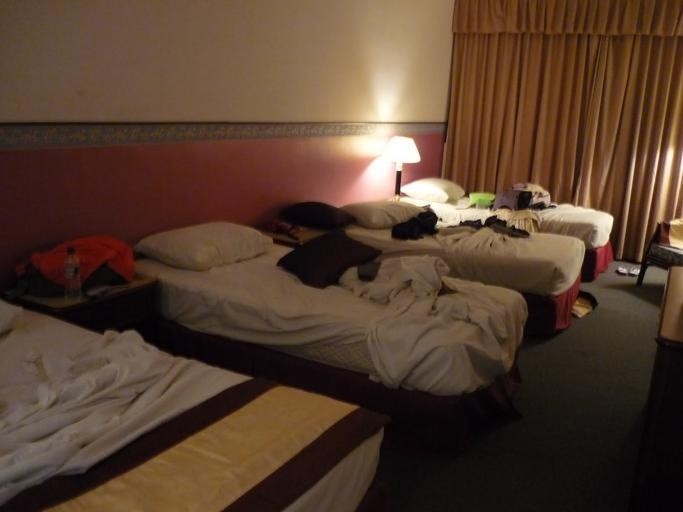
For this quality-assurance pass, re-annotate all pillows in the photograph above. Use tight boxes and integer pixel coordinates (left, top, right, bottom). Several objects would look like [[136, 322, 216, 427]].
[[273, 228, 383, 291], [401, 178, 467, 202], [340, 201, 425, 230], [132, 221, 273, 275], [285, 200, 355, 229], [0, 298, 25, 334]]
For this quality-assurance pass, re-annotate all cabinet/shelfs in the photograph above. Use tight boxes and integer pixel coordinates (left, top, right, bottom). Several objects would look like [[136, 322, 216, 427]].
[[630, 264, 683, 512]]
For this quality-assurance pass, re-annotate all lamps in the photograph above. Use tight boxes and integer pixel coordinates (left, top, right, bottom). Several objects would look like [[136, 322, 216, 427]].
[[379, 137, 421, 199]]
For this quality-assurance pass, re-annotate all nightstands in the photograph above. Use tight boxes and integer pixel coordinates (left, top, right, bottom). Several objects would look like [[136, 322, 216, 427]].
[[4, 272, 160, 345], [266, 219, 328, 246]]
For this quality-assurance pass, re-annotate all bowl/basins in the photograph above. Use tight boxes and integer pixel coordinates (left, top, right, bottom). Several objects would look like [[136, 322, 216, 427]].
[[468, 193, 495, 208]]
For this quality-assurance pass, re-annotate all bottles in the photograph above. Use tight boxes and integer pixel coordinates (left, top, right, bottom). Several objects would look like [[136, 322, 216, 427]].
[[64, 247, 81, 303]]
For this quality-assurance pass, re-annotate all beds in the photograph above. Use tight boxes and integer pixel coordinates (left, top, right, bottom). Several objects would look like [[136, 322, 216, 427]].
[[335, 200, 584, 343], [399, 172, 617, 282], [0, 298, 390, 510], [132, 218, 532, 447]]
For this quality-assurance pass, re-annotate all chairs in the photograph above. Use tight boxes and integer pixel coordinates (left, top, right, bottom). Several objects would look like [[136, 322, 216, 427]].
[[635, 219, 683, 285]]
